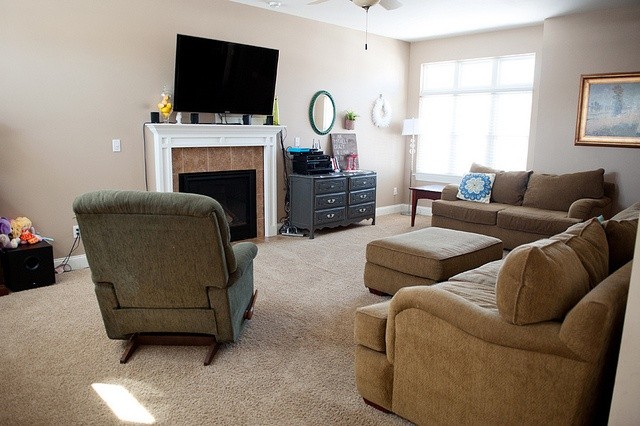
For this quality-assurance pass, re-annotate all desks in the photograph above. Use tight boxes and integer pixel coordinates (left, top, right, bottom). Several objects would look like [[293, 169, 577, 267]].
[[409, 185, 446, 227]]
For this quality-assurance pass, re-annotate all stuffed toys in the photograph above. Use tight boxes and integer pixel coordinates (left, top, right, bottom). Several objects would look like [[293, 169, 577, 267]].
[[17, 216, 38, 243], [0, 219, 22, 248]]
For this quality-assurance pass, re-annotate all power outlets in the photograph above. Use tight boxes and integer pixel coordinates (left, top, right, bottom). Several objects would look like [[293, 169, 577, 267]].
[[73, 226, 82, 238], [393, 186, 398, 195]]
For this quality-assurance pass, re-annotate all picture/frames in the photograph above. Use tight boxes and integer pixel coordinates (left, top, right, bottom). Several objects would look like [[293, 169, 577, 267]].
[[574, 71, 640, 148]]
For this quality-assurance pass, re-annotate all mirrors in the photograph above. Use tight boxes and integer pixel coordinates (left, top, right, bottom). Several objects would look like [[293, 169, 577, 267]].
[[308, 89, 336, 136]]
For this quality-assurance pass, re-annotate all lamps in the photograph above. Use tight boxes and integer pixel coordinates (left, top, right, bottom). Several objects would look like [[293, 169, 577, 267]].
[[401, 119, 420, 216], [350, 0, 380, 50]]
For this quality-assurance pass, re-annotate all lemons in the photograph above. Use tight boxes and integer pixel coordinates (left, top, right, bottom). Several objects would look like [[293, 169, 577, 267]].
[[158, 96, 172, 118]]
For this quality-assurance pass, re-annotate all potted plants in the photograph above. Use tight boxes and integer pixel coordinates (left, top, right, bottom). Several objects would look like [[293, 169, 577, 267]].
[[344, 111, 360, 130]]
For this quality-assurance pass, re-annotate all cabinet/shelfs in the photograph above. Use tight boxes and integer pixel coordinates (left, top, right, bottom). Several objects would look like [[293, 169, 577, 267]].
[[348, 170, 377, 225], [288, 172, 348, 239]]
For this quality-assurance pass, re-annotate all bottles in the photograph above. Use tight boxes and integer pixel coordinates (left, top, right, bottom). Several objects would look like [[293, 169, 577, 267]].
[[273, 98, 279, 123]]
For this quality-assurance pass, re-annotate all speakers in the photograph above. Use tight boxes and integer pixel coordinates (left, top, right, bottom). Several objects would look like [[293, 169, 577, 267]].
[[0, 239, 56, 292]]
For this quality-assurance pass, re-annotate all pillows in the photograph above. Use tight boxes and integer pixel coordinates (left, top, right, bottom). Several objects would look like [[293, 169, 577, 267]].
[[601, 201, 639, 260], [523, 168, 605, 212], [456, 173, 496, 204], [495, 218, 609, 326], [469, 163, 533, 206]]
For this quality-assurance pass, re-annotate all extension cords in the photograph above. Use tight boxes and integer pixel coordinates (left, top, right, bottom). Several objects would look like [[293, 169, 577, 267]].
[[281, 232, 305, 237]]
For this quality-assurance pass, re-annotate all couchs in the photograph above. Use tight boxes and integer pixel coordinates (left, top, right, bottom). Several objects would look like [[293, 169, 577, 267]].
[[364, 227, 503, 297], [431, 182, 619, 250], [355, 259, 632, 426], [72, 189, 258, 366]]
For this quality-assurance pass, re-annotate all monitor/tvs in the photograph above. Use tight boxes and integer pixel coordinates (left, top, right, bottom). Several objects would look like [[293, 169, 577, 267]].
[[173, 33, 279, 125]]
